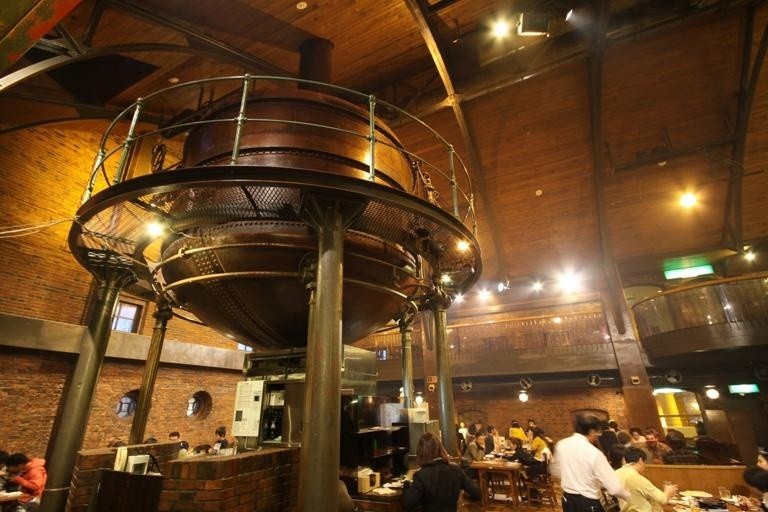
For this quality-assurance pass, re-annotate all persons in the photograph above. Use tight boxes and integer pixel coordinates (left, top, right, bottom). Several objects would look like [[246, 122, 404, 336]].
[[0, 451, 48, 512], [401, 432, 482, 512], [742, 451, 768, 512], [547, 414, 678, 512], [456, 418, 555, 504], [664, 430, 732, 465], [107, 427, 240, 454]]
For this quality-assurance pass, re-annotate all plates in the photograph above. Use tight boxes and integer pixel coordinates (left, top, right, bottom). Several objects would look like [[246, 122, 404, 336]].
[[680, 490, 712, 498], [383, 482, 403, 488]]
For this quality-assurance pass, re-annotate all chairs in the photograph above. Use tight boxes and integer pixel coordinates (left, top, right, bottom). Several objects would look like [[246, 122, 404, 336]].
[[527, 460, 559, 510]]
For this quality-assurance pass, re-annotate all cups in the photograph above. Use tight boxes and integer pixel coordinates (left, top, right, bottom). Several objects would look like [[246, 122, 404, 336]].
[[661, 480, 672, 486], [717, 486, 730, 498]]
